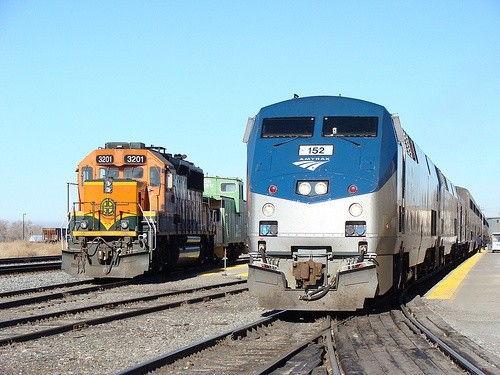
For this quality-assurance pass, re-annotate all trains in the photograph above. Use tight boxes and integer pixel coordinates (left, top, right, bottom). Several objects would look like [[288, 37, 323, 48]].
[[61, 142, 248, 284], [241, 94, 489, 311]]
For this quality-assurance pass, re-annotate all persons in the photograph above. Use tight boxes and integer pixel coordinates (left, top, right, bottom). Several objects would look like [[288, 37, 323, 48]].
[[477, 235, 483, 253], [483, 235, 487, 250]]
